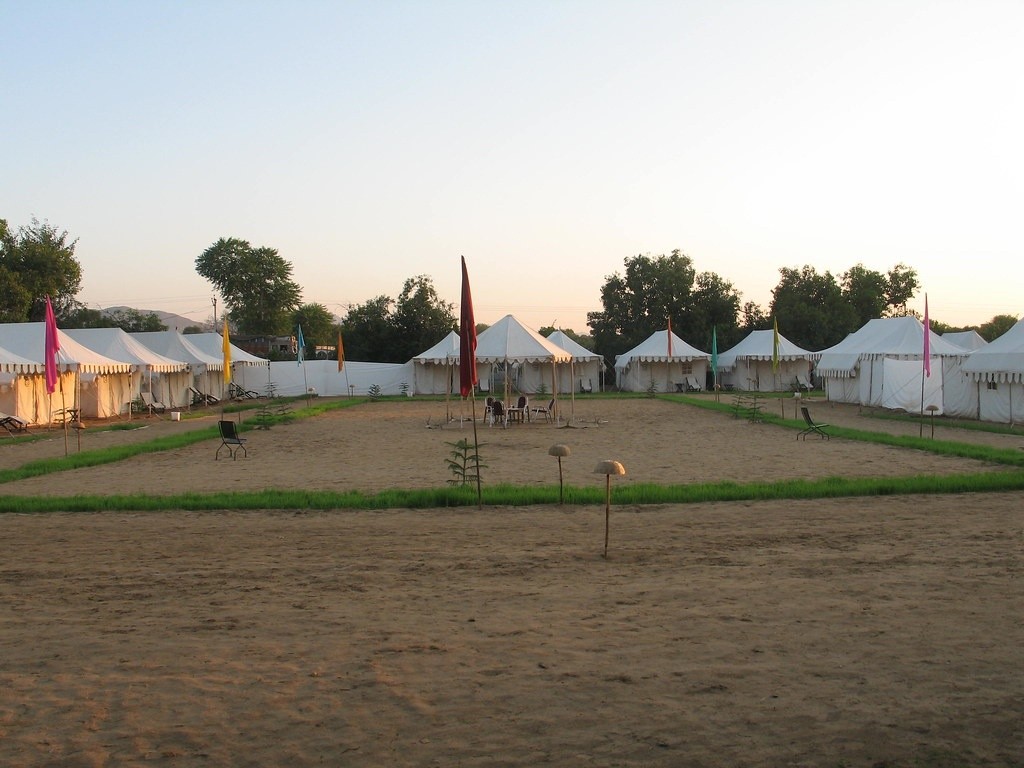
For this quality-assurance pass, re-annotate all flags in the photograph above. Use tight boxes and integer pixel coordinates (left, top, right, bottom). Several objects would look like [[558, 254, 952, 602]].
[[667, 317, 672, 358], [459, 256, 478, 398], [712, 326, 718, 375], [923, 295, 931, 377], [337, 331, 345, 373], [45, 296, 61, 394], [222, 318, 233, 386], [773, 316, 780, 373], [297, 323, 306, 367]]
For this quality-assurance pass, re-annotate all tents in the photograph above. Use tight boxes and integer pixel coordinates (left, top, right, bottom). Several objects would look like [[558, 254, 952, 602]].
[[414, 315, 605, 395], [0, 323, 271, 438], [615, 318, 1024, 426]]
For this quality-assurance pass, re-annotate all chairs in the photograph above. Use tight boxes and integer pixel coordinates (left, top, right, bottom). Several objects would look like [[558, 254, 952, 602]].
[[796, 375, 814, 392], [532, 400, 555, 423], [190, 387, 221, 407], [216, 421, 247, 461], [0, 412, 33, 439], [484, 396, 530, 428], [580, 378, 592, 392], [796, 407, 829, 441], [686, 376, 702, 393], [231, 382, 259, 399], [140, 393, 164, 414]]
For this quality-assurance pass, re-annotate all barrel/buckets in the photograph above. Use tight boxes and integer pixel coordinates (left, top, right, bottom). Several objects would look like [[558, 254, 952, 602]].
[[170, 411, 181, 421]]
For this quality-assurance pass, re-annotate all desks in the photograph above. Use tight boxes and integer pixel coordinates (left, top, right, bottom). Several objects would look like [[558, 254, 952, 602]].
[[675, 383, 683, 393], [68, 409, 82, 424], [507, 407, 524, 424], [724, 384, 733, 392]]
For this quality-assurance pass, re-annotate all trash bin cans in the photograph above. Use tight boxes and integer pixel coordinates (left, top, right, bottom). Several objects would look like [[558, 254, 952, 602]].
[[170, 411, 181, 421], [794, 392, 802, 399], [405, 390, 413, 397]]
